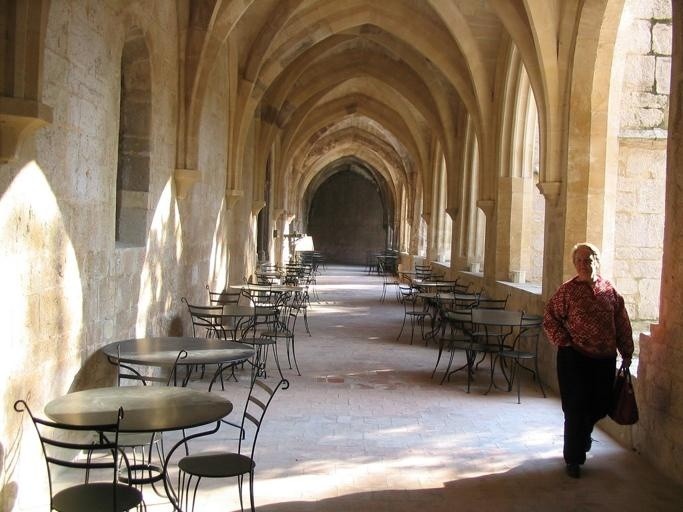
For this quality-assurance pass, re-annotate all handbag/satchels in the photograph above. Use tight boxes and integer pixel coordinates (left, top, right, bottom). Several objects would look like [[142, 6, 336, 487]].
[[607, 376, 639, 425]]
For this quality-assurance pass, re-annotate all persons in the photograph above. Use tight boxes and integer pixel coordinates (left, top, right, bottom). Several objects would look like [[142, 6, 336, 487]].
[[542, 242, 635, 479]]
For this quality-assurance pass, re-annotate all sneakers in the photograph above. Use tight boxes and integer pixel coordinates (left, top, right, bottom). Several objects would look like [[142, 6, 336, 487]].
[[562, 457, 580, 478]]
